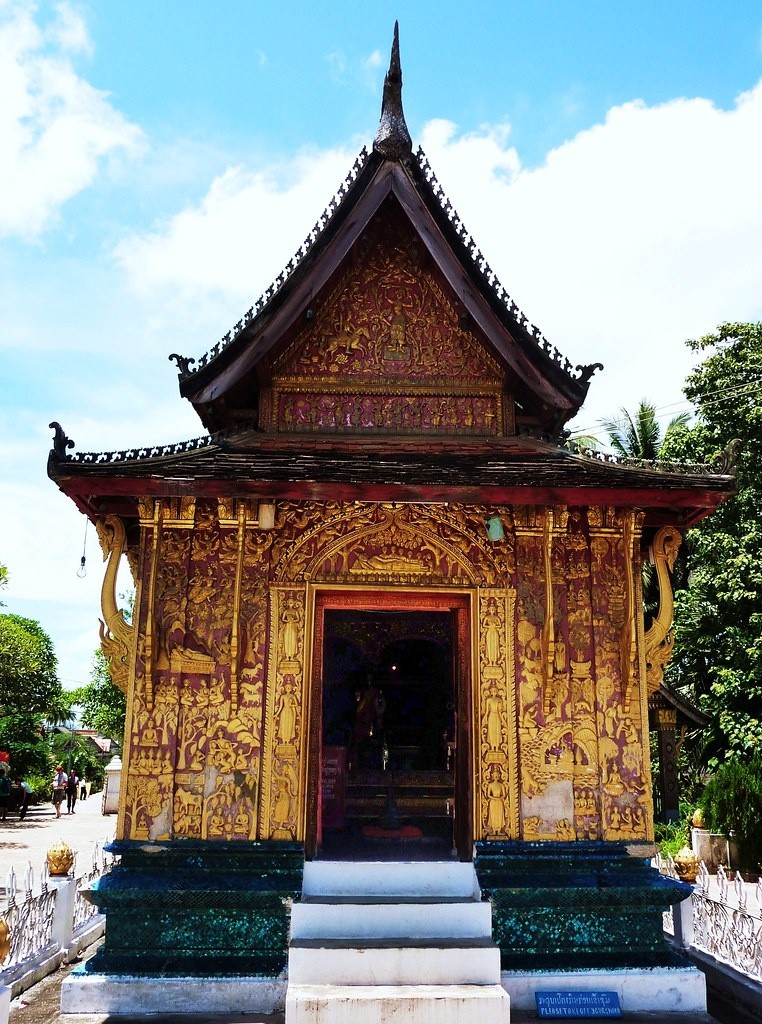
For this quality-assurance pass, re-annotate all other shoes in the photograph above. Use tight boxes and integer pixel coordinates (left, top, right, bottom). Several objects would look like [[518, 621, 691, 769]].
[[0, 818, 7, 821], [66, 811, 76, 814], [19, 816, 25, 822], [80, 798, 87, 801], [55, 814, 61, 818]]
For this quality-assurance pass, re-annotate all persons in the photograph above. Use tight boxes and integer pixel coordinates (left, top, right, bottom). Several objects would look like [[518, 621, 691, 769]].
[[384, 284, 413, 354], [483, 606, 501, 667], [282, 599, 299, 662], [483, 688, 504, 753], [15, 778, 33, 820], [52, 766, 68, 819], [487, 772, 506, 835], [66, 770, 79, 814], [271, 758, 295, 830], [0, 769, 9, 821], [80, 777, 87, 800], [274, 684, 298, 746]]
